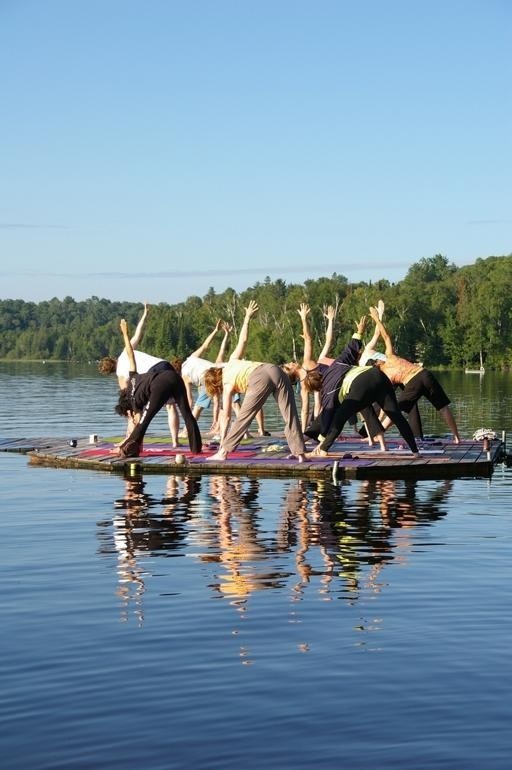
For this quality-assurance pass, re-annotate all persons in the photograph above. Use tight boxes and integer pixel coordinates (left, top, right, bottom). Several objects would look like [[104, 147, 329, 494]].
[[276, 303, 391, 452], [361, 307, 462, 446], [203, 299, 311, 463], [96, 300, 183, 450], [303, 314, 423, 458], [356, 300, 425, 440], [214, 321, 271, 436], [114, 317, 204, 457], [312, 306, 375, 448], [170, 317, 252, 439]]
